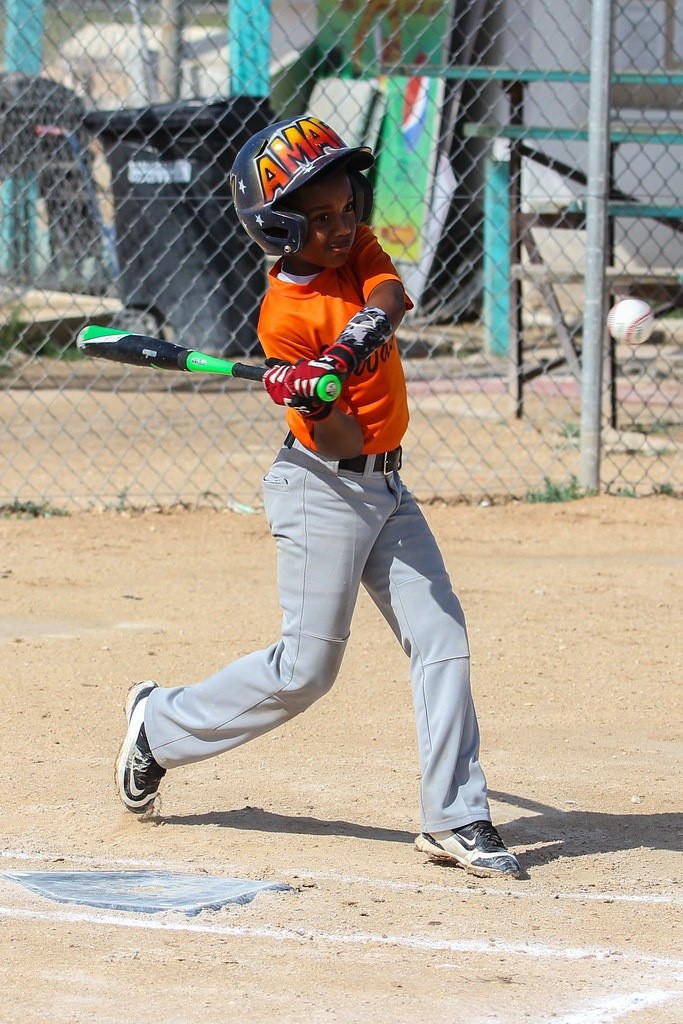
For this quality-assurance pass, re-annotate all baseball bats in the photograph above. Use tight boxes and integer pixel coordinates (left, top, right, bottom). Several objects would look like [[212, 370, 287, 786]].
[[75, 325, 344, 403]]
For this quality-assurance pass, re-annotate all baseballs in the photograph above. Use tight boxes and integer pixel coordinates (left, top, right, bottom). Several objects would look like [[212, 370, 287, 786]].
[[606, 297, 655, 346]]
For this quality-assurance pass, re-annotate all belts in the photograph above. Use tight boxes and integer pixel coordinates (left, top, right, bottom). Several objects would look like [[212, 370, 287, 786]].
[[286, 432, 404, 475]]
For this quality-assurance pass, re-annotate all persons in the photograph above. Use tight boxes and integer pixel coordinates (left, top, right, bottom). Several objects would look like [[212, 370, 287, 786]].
[[112, 113, 525, 881]]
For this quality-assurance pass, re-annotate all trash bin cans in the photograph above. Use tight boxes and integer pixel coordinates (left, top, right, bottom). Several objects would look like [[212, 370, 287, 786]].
[[83, 96, 273, 357]]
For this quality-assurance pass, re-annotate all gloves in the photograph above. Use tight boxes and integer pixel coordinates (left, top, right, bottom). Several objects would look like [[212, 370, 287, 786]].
[[285, 344, 359, 399], [261, 358, 334, 424]]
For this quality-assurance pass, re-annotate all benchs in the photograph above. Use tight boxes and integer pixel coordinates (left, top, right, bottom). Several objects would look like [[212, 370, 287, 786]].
[[412, 63, 683, 425]]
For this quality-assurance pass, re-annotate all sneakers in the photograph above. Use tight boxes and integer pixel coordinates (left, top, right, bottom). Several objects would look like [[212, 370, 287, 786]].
[[115, 681, 166, 814], [415, 819, 521, 879]]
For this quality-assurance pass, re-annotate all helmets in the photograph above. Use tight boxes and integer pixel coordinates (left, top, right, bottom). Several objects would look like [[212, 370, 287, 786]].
[[229, 115, 377, 256]]
